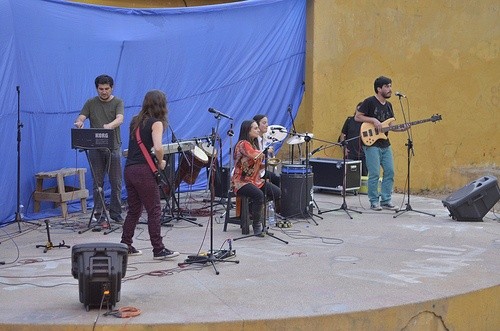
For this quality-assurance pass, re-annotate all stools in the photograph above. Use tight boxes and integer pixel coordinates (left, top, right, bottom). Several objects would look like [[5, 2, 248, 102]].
[[224, 192, 266, 235]]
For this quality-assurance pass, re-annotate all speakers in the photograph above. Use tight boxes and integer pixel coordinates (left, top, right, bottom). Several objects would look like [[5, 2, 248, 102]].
[[442, 176, 500, 222], [280, 173, 314, 220], [71, 243, 128, 310]]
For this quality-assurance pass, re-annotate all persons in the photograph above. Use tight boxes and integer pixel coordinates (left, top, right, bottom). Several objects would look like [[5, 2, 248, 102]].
[[120, 90, 180, 260], [74, 75, 125, 229], [234, 120, 281, 237], [354, 75, 399, 211], [340, 102, 368, 177], [251, 114, 281, 214]]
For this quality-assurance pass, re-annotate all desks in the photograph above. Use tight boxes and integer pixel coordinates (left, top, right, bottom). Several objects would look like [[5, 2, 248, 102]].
[[33, 167, 89, 218]]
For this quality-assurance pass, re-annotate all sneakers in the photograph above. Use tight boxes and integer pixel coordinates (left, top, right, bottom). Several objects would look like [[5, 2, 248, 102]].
[[128, 245, 142, 255], [382, 203, 398, 209], [154, 248, 179, 259], [371, 202, 382, 210]]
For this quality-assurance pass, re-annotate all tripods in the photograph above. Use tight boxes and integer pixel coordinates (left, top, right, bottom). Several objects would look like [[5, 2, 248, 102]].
[[394, 96, 435, 218], [0, 86, 42, 233], [161, 121, 362, 275]]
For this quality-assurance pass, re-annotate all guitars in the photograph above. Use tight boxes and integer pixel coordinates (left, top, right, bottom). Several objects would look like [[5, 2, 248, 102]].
[[360, 113, 442, 147], [156, 167, 171, 197]]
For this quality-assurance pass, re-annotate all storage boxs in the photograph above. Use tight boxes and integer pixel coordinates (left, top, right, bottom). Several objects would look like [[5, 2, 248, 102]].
[[309, 158, 363, 196]]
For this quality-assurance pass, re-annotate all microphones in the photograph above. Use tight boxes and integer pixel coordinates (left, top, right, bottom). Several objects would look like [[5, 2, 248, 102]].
[[208, 107, 233, 120], [267, 134, 282, 142], [395, 92, 407, 98], [308, 146, 323, 156], [271, 127, 283, 130], [287, 107, 291, 112]]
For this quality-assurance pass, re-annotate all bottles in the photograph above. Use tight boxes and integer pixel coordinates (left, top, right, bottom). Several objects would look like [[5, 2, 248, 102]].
[[19, 203, 25, 218], [269, 200, 275, 222]]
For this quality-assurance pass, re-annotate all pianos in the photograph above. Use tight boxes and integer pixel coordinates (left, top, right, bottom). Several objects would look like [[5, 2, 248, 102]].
[[122, 136, 213, 225]]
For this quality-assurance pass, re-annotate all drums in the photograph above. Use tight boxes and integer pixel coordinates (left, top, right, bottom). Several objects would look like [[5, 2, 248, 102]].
[[201, 142, 217, 168], [179, 146, 208, 184]]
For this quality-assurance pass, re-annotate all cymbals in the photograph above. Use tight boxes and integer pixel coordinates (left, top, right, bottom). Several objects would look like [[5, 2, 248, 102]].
[[262, 125, 288, 144], [287, 133, 313, 144]]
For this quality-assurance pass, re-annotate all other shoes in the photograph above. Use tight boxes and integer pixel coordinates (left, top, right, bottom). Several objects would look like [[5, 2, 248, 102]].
[[97, 217, 105, 224], [252, 223, 262, 235], [111, 215, 124, 222]]
